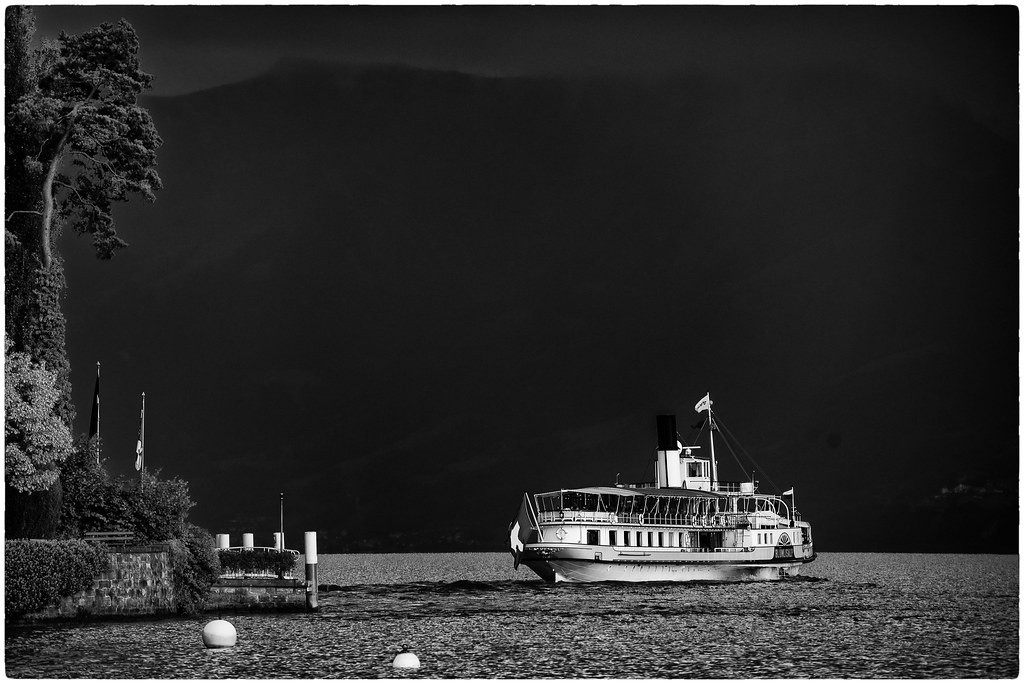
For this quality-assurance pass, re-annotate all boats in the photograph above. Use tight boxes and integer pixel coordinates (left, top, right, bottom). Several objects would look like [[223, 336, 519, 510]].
[[509, 414, 818, 583]]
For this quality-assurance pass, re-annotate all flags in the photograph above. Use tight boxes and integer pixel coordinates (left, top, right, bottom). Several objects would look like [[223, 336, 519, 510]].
[[134, 399, 144, 472], [783, 488, 793, 496], [89, 368, 98, 442], [695, 395, 709, 413]]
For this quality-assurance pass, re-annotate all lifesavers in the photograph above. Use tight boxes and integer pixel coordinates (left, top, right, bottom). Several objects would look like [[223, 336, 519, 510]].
[[702, 515, 707, 527], [609, 512, 616, 524], [692, 516, 696, 527], [711, 517, 716, 527], [638, 513, 645, 524], [555, 527, 568, 540]]
